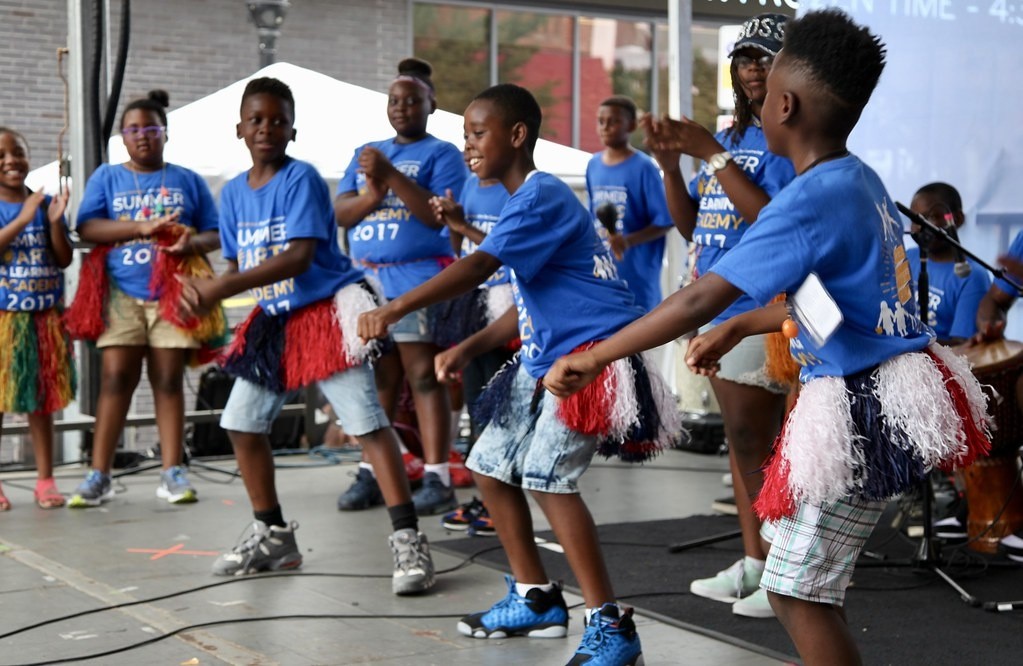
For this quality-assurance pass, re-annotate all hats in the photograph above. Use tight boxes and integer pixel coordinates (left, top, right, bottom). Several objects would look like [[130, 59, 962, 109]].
[[726, 13, 793, 58]]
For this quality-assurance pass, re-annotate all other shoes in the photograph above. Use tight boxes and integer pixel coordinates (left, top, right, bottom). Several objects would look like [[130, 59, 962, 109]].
[[448, 451, 474, 486], [905, 492, 938, 537], [713, 496, 738, 515], [401, 453, 425, 479], [1000, 534, 1023, 561], [933, 517, 967, 538]]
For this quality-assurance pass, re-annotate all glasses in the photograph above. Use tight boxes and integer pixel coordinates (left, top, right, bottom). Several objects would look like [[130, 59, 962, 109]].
[[120, 126, 165, 140], [734, 54, 773, 71]]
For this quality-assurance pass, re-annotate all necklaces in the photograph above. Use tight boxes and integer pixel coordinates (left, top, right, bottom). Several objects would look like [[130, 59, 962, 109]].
[[130, 162, 165, 225]]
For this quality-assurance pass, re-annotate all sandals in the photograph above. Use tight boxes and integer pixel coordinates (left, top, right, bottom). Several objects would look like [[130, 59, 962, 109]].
[[34, 478, 65, 509]]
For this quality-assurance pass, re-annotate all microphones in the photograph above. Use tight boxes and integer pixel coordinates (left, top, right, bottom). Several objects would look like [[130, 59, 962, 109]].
[[940, 202, 971, 276]]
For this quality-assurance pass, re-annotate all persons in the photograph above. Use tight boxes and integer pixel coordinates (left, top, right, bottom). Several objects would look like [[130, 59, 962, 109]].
[[933, 226, 1023, 561], [641, 16, 799, 618], [0, 125, 74, 512], [334, 57, 470, 521], [164, 76, 436, 598], [584, 95, 677, 320], [544, 7, 995, 666], [67, 89, 221, 507], [428, 168, 516, 536], [899, 182, 992, 348], [358, 83, 646, 666]]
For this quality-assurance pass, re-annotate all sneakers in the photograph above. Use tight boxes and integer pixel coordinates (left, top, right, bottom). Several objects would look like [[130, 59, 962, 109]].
[[441, 495, 499, 536], [212, 519, 303, 576], [566, 603, 645, 666], [457, 575, 569, 639], [156, 465, 199, 503], [732, 590, 778, 617], [411, 472, 458, 514], [690, 555, 767, 603], [387, 529, 436, 594], [337, 462, 385, 510], [67, 469, 115, 507]]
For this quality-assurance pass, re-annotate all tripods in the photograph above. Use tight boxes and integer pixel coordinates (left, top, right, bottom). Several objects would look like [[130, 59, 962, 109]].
[[112, 449, 243, 479], [851, 200, 1022, 608]]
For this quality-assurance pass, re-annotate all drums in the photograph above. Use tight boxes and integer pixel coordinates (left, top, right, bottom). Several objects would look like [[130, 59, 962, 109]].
[[949, 339, 1023, 555]]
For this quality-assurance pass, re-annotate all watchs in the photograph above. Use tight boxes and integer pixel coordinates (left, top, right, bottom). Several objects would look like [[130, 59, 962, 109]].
[[706, 151, 734, 175]]
